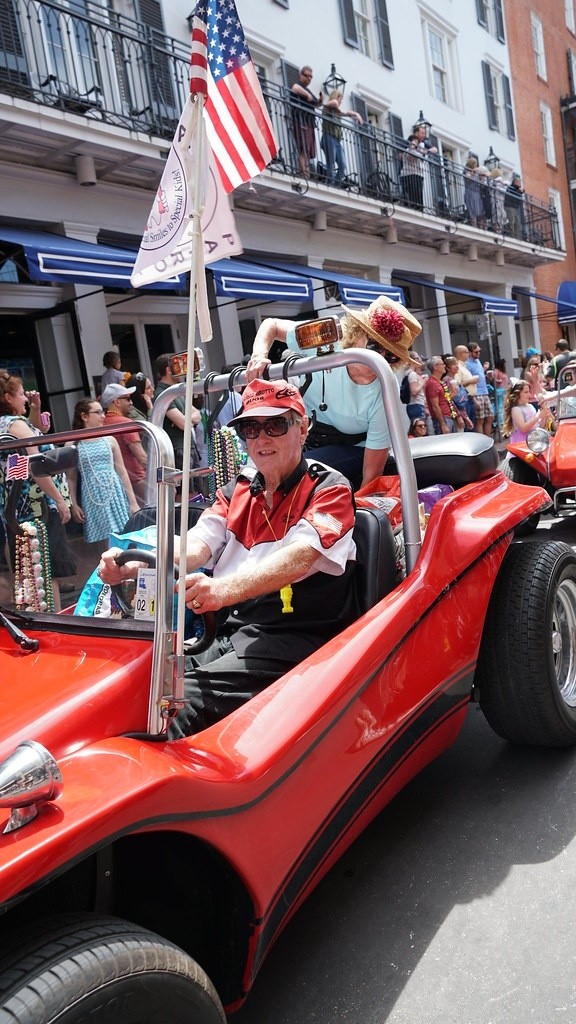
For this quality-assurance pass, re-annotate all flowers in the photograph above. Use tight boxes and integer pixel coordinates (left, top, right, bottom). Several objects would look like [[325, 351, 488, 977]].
[[370, 307, 406, 343]]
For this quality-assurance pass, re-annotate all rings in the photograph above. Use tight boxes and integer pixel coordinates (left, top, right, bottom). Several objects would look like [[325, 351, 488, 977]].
[[193, 600, 202, 609], [97, 570, 102, 577]]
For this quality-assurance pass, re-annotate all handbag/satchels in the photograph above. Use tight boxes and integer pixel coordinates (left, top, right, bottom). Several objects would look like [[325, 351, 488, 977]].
[[453, 384, 469, 409]]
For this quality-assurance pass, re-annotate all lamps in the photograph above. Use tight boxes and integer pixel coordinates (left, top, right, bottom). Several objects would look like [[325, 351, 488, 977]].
[[467, 245, 478, 261], [323, 63, 347, 98], [228, 192, 234, 211], [314, 211, 327, 231], [485, 146, 500, 172], [74, 155, 98, 186], [440, 241, 450, 255], [387, 228, 398, 244], [413, 110, 432, 141], [186, 0, 199, 29], [496, 251, 504, 266]]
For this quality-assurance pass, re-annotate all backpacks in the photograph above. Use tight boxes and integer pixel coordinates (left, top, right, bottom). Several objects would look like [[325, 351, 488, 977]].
[[400, 371, 412, 404]]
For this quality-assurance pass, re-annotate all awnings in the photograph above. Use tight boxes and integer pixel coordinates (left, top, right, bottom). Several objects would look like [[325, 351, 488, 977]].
[[0, 226, 187, 291], [394, 274, 519, 318], [556, 280, 576, 326], [107, 241, 313, 302], [231, 254, 406, 307], [513, 287, 576, 310]]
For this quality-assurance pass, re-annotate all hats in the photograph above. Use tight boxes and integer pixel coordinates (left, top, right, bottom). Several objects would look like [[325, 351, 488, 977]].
[[226, 376, 306, 429], [101, 383, 137, 407], [340, 295, 423, 368]]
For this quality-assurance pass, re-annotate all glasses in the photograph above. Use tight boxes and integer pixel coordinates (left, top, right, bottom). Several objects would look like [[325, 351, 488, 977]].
[[472, 349, 482, 353], [112, 397, 131, 403], [136, 371, 144, 386], [415, 425, 427, 428], [86, 410, 106, 416], [237, 416, 304, 439], [365, 333, 400, 364], [531, 363, 540, 366]]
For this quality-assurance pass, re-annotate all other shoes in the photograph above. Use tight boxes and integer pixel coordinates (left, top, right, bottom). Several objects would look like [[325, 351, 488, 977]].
[[56, 580, 75, 592]]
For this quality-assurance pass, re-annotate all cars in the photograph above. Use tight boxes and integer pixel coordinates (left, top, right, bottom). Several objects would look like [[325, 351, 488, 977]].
[[496, 360, 576, 540], [0, 315, 576, 1024]]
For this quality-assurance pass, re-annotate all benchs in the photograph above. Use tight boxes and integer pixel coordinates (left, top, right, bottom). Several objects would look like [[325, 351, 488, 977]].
[[361, 431, 501, 485]]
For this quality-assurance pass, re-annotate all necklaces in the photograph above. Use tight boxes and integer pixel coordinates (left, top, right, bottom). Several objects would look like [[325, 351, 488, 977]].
[[12, 518, 56, 613], [107, 410, 123, 416], [432, 375, 458, 419], [205, 425, 248, 506], [261, 474, 305, 615]]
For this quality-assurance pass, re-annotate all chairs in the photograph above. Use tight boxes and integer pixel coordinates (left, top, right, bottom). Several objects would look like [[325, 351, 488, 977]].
[[526, 228, 556, 248], [439, 200, 473, 226], [122, 103, 180, 139], [292, 152, 362, 194], [123, 502, 400, 620], [34, 75, 106, 121], [268, 147, 287, 172], [366, 171, 410, 208]]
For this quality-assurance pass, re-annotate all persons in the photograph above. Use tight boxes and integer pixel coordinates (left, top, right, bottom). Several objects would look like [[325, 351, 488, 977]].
[[242, 295, 424, 491], [290, 64, 362, 187], [498, 339, 576, 487], [98, 351, 246, 502], [396, 126, 438, 212], [61, 395, 142, 542], [100, 382, 148, 510], [98, 377, 367, 741], [463, 157, 526, 240], [390, 340, 509, 440], [0, 369, 77, 613]]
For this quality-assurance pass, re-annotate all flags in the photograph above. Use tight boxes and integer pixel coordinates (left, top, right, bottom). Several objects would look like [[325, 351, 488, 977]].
[[128, 93, 247, 288], [186, 0, 282, 198]]
